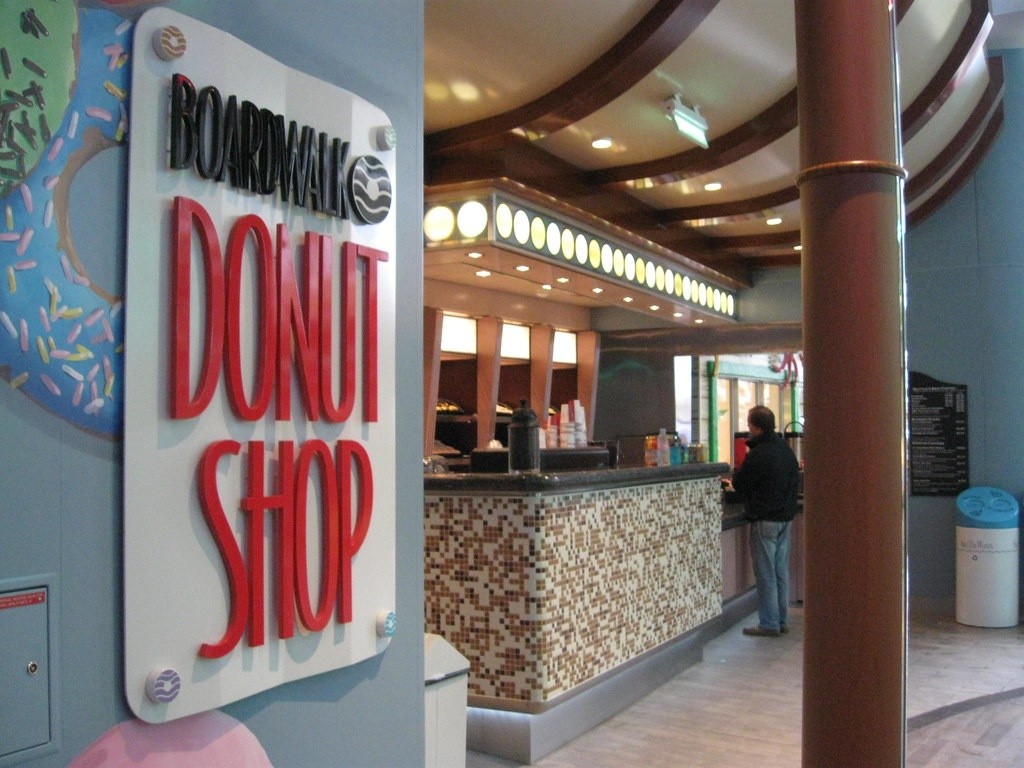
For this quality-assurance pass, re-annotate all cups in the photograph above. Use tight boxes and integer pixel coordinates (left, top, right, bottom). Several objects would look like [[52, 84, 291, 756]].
[[549, 400, 587, 449]]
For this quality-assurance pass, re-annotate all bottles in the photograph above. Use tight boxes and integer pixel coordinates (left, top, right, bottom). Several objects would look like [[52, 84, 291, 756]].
[[670, 432, 681, 465], [656, 428, 670, 466], [508, 399, 540, 473], [689, 440, 702, 462], [681, 445, 689, 464]]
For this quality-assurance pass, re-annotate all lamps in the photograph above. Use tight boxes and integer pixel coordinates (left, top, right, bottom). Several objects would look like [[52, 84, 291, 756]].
[[662, 93, 710, 149]]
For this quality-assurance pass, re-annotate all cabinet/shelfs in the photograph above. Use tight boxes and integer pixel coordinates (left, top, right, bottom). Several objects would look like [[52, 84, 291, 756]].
[[429, 411, 515, 466]]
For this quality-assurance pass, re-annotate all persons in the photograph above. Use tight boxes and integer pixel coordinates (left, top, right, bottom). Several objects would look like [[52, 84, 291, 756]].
[[721, 405, 801, 637]]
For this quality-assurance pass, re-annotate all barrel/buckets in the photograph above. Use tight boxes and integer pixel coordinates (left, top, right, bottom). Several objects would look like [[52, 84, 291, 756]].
[[734, 432, 751, 470], [784, 421, 804, 468]]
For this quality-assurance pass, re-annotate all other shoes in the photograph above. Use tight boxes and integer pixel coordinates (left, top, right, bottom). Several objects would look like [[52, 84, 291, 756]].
[[780, 627, 788, 633], [743, 627, 778, 636]]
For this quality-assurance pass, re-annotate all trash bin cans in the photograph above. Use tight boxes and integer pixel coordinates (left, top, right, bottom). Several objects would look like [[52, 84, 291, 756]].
[[952, 487, 1019, 628]]
